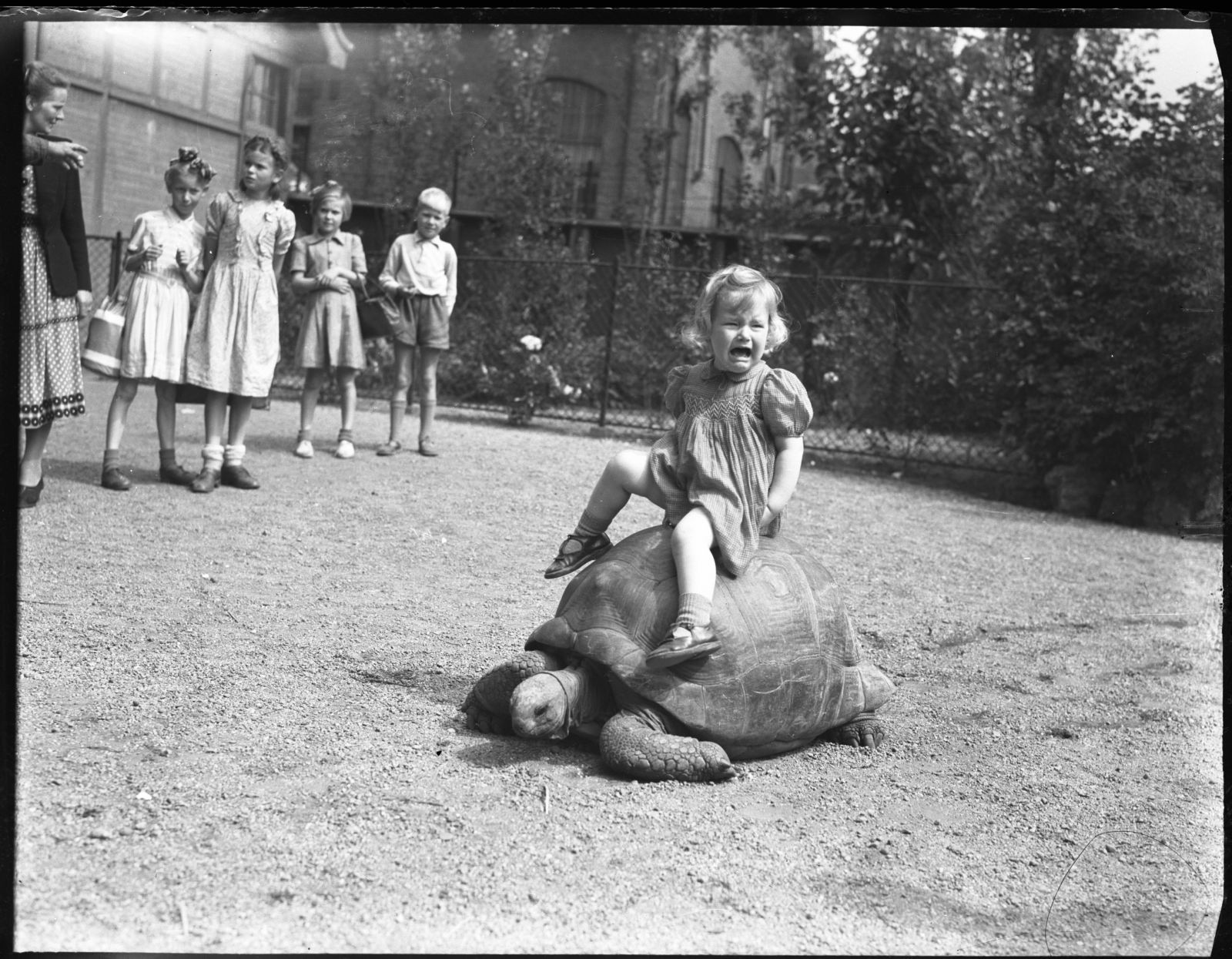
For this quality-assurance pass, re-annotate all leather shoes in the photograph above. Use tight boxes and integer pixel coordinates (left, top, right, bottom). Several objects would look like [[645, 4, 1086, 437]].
[[220, 465, 261, 487], [646, 622, 722, 669], [101, 468, 131, 488], [192, 468, 222, 491], [544, 531, 614, 579], [160, 466, 199, 485]]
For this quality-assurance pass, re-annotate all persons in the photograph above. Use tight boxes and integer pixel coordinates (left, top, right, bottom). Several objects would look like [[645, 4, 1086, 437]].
[[98, 146, 205, 491], [24, 130, 92, 171], [290, 183, 368, 459], [377, 185, 459, 455], [545, 263, 813, 669], [185, 134, 297, 493], [20, 63, 94, 508]]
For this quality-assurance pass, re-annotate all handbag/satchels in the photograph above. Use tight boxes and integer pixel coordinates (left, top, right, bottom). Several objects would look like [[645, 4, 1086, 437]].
[[356, 271, 405, 338], [81, 255, 142, 378]]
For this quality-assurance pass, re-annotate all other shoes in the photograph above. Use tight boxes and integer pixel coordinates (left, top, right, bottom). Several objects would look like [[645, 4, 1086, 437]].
[[378, 438, 403, 454], [18, 477, 44, 508], [419, 437, 439, 455], [297, 441, 314, 457], [336, 441, 355, 458]]
